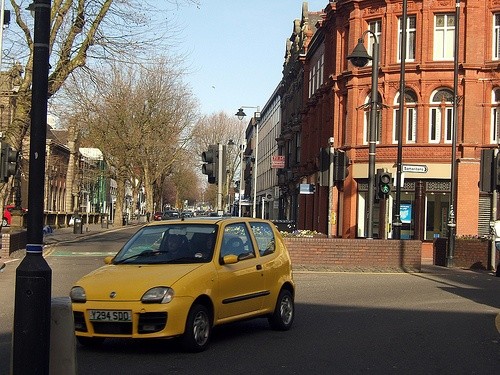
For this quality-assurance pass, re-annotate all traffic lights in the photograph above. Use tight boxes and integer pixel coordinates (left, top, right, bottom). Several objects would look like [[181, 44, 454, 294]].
[[333, 150, 349, 181], [0, 143, 19, 183], [201, 148, 216, 179], [308, 184, 316, 192], [375, 168, 391, 199]]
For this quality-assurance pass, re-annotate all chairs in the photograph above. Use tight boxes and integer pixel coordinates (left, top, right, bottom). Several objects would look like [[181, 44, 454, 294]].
[[226, 237, 244, 251], [165, 234, 188, 251]]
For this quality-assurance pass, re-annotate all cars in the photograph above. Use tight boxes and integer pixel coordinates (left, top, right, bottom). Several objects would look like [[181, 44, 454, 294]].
[[69, 213, 296, 353], [153, 206, 220, 221]]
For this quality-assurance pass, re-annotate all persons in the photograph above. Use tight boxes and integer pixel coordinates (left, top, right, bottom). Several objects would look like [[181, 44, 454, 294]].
[[146, 211, 151, 222]]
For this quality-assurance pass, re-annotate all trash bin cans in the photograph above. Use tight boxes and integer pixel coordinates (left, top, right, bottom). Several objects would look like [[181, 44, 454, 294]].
[[74, 218, 82, 233], [123, 215, 127, 225]]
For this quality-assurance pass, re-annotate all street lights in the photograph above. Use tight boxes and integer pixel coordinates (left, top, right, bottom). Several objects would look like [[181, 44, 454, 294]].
[[345, 29, 379, 239], [235, 105, 260, 218]]
